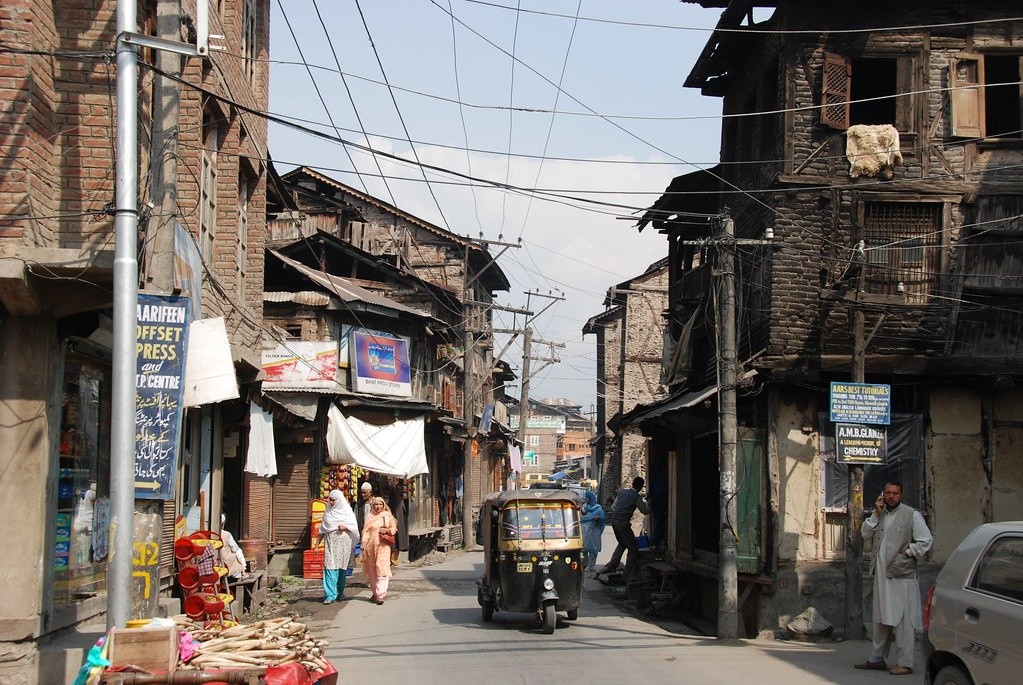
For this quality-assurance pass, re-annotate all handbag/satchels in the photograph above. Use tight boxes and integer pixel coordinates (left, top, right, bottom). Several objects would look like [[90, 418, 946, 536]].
[[635, 530, 650, 548], [379, 516, 395, 545]]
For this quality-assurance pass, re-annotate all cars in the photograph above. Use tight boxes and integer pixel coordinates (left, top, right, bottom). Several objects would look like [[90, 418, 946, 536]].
[[922, 520, 1023, 685]]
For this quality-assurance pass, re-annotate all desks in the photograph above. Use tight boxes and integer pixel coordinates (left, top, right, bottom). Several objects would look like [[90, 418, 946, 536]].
[[83, 617, 337, 685]]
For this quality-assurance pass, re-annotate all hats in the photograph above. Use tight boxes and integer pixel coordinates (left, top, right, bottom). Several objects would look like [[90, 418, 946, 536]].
[[361, 482, 372, 491]]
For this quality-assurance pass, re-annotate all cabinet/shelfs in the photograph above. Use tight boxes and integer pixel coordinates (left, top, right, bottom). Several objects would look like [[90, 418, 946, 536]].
[[172, 514, 241, 630], [309, 499, 328, 550]]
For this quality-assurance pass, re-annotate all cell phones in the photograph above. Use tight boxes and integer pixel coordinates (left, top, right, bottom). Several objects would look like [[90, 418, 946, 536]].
[[881, 491, 884, 503]]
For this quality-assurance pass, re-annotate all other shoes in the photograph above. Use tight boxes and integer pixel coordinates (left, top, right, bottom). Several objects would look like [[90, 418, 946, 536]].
[[322, 600, 331, 604], [376, 601, 384, 605], [889, 665, 913, 675], [338, 596, 345, 602], [586, 568, 595, 572], [854, 659, 887, 669], [369, 594, 376, 601]]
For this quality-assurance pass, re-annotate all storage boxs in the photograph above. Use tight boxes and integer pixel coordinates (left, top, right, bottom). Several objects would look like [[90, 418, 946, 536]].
[[303, 550, 330, 578]]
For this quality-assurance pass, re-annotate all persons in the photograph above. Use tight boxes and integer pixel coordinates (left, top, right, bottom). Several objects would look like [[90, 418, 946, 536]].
[[221, 514, 248, 579], [606, 477, 650, 575], [318, 483, 397, 605], [579, 491, 605, 572], [855, 482, 934, 674]]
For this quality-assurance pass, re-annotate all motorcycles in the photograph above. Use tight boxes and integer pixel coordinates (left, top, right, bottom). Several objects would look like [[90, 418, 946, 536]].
[[475, 489, 587, 634]]
